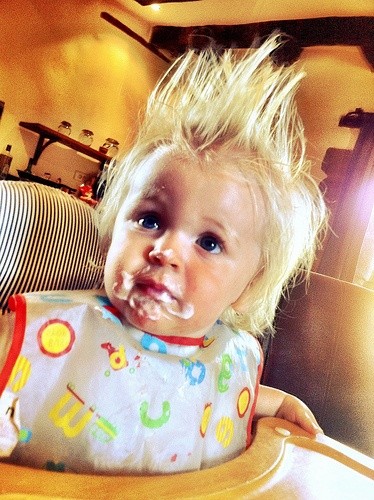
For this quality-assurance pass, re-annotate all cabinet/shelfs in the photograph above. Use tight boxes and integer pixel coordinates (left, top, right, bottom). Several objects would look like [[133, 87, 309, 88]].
[[17, 121, 115, 200]]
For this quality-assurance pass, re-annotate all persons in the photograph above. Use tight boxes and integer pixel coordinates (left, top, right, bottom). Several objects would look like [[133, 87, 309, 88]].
[[0, 34, 331, 477]]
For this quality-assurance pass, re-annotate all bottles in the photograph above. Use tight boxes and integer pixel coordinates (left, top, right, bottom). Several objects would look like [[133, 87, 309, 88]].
[[21, 157, 33, 182], [0, 144, 14, 181]]
